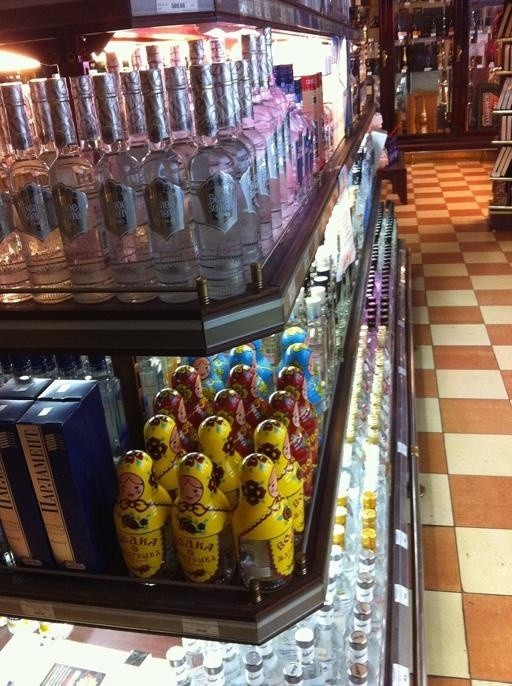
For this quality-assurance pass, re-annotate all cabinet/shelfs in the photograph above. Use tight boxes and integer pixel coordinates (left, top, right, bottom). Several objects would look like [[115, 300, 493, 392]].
[[0, 0, 425, 686], [487, 0, 512, 235]]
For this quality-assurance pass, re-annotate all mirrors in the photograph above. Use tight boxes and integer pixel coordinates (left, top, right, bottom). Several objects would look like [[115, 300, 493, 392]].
[[377, 0, 470, 142]]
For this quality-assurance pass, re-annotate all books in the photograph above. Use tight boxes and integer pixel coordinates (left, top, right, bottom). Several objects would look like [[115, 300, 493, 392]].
[[487, 1, 511, 208]]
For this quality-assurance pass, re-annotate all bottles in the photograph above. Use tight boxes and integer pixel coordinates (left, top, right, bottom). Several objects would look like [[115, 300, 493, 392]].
[[409, 15, 420, 39], [401, 46, 409, 73], [395, 12, 408, 41], [474, 7, 493, 34], [0, 24, 393, 686], [418, 95, 428, 134], [429, 15, 437, 37]]
